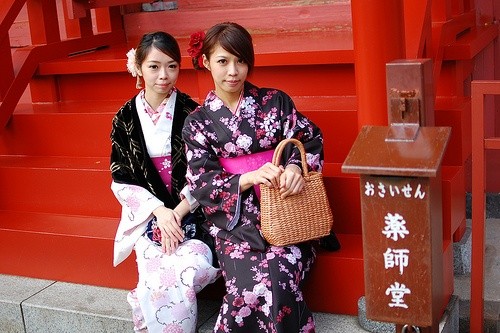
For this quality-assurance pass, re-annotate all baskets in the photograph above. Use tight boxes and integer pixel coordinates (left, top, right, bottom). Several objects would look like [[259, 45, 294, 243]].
[[259, 138, 333, 247]]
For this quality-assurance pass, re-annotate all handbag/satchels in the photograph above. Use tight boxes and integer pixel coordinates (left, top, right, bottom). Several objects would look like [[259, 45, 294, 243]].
[[145, 213, 196, 247]]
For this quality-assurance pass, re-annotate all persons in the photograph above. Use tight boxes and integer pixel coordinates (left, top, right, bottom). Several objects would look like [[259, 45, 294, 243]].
[[110, 31, 219, 333], [183, 22, 341, 333]]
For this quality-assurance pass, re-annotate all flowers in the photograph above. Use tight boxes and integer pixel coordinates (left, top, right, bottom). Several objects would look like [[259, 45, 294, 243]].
[[126, 48, 143, 77], [187, 29, 206, 58]]
